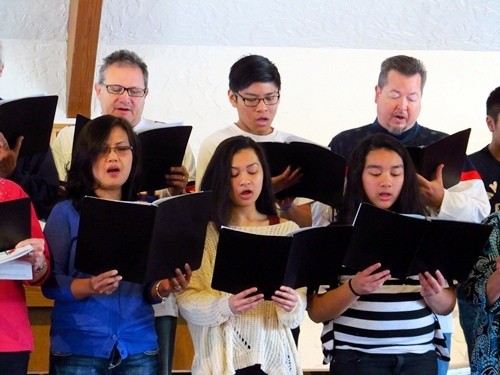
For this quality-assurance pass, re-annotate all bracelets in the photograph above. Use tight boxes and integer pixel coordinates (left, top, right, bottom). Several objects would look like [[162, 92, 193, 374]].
[[156, 279, 169, 301], [38, 260, 46, 271], [275, 202, 294, 210]]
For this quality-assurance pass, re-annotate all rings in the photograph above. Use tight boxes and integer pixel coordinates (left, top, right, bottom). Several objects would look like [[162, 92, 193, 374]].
[[175, 285, 181, 291]]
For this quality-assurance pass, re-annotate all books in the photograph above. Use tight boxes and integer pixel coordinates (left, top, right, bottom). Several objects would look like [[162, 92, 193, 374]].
[[66, 114, 194, 194], [75, 190, 208, 283], [209, 203, 496, 301], [256, 127, 472, 205], [0, 197, 32, 279], [0, 95, 58, 160]]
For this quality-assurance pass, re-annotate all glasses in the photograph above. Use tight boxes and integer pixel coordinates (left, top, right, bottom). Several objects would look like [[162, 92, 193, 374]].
[[235, 91, 280, 107], [100, 82, 146, 97], [98, 146, 132, 158]]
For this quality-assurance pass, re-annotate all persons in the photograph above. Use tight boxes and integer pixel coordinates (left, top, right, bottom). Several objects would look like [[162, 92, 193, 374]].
[[52, 49, 197, 203], [326, 55, 491, 224], [176, 135, 306, 375], [467, 88, 500, 213], [194, 55, 323, 228], [458, 212, 500, 375], [42, 117, 192, 375], [306, 132, 457, 375], [0, 133, 51, 375], [0, 40, 6, 80]]
